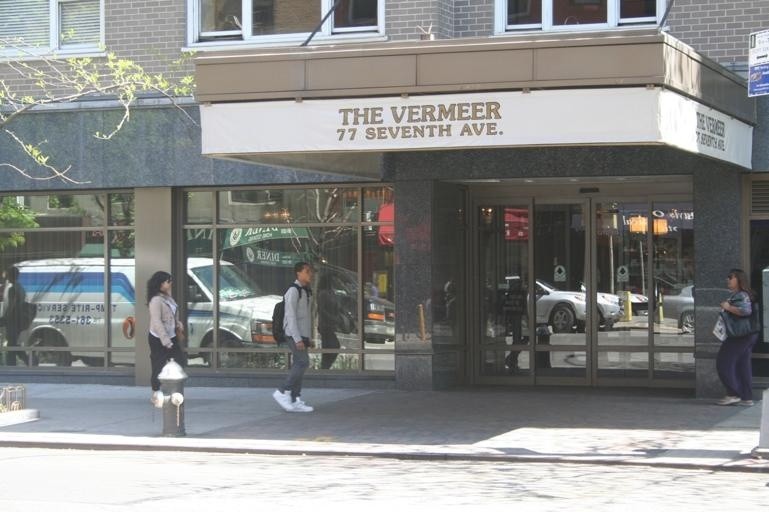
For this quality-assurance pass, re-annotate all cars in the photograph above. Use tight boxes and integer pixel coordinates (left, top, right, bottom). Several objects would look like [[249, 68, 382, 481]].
[[654, 285, 694, 331], [321, 263, 396, 344], [496, 273, 625, 334]]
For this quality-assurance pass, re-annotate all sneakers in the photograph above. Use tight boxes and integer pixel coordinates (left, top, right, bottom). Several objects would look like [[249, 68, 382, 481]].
[[716, 394, 754, 406], [273, 389, 313, 412]]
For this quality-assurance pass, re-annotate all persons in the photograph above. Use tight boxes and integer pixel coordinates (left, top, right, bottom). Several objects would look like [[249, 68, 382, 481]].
[[366, 280, 378, 298], [3, 267, 28, 366], [717, 269, 761, 406], [505, 267, 529, 373], [272, 262, 315, 412], [444, 276, 456, 320], [317, 274, 344, 369], [146, 271, 184, 402]]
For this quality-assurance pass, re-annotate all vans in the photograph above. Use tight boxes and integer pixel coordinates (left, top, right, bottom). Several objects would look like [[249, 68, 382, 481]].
[[4, 256, 292, 369]]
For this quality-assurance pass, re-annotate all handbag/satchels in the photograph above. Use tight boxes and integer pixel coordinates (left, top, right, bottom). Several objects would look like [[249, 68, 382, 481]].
[[719, 302, 760, 338], [176, 328, 185, 342], [273, 302, 284, 343]]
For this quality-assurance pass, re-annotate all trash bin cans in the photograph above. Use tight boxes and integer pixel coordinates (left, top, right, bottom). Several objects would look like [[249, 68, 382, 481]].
[[616, 290, 632, 322], [535, 325, 553, 369]]
